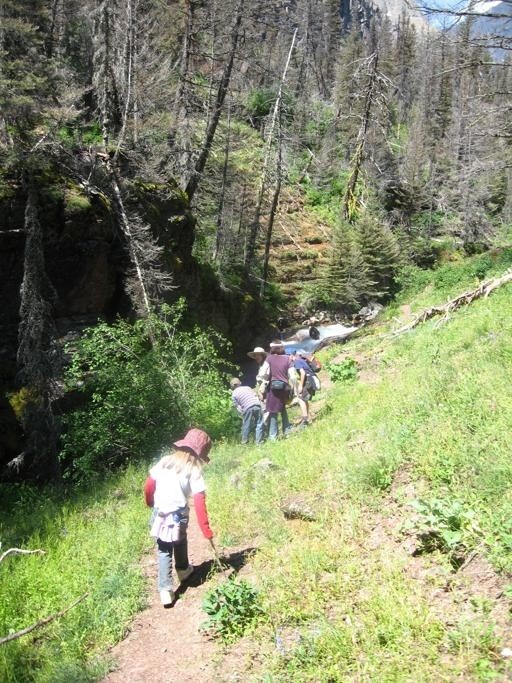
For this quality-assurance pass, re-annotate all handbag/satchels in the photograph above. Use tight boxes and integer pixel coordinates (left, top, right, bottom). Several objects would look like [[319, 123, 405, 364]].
[[307, 373, 321, 389], [270, 380, 285, 391]]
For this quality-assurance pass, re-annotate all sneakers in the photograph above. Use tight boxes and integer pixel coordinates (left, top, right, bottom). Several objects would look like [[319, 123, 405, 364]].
[[177, 565, 194, 581], [160, 591, 175, 605]]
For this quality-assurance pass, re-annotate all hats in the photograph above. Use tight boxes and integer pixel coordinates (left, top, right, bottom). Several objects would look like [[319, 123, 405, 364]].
[[285, 348, 295, 355], [247, 347, 268, 358], [173, 429, 212, 464]]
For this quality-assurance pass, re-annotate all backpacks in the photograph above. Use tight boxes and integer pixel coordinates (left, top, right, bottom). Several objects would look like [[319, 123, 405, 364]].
[[306, 356, 321, 372]]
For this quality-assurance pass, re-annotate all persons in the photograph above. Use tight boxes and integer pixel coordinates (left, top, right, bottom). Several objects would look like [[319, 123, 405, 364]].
[[247, 341, 321, 443], [144, 428, 214, 605], [230, 378, 264, 446]]
